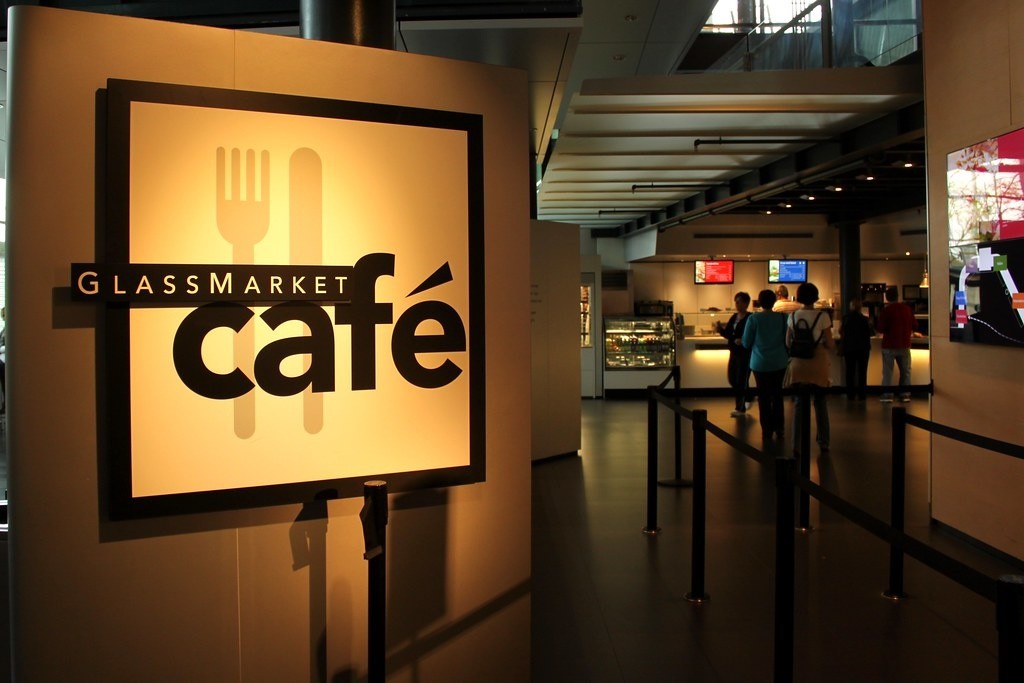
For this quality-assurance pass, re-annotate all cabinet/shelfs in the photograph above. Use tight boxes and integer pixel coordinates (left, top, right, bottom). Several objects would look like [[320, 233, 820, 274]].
[[602, 314, 677, 399]]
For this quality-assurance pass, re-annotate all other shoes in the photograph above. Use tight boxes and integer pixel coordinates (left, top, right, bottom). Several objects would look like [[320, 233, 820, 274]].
[[879, 393, 894, 403], [820, 447, 830, 451], [730, 409, 746, 416], [853, 399, 866, 406], [744, 395, 755, 409], [900, 392, 912, 402], [792, 449, 801, 460]]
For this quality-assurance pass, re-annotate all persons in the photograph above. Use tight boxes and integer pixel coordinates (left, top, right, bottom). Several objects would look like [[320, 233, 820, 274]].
[[877, 288, 919, 403], [785, 284, 840, 458], [742, 288, 789, 440], [836, 299, 876, 413], [868, 300, 884, 334], [716, 291, 755, 416]]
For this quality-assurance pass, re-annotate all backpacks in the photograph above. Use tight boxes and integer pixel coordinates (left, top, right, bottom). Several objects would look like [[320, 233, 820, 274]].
[[788, 312, 824, 359]]
